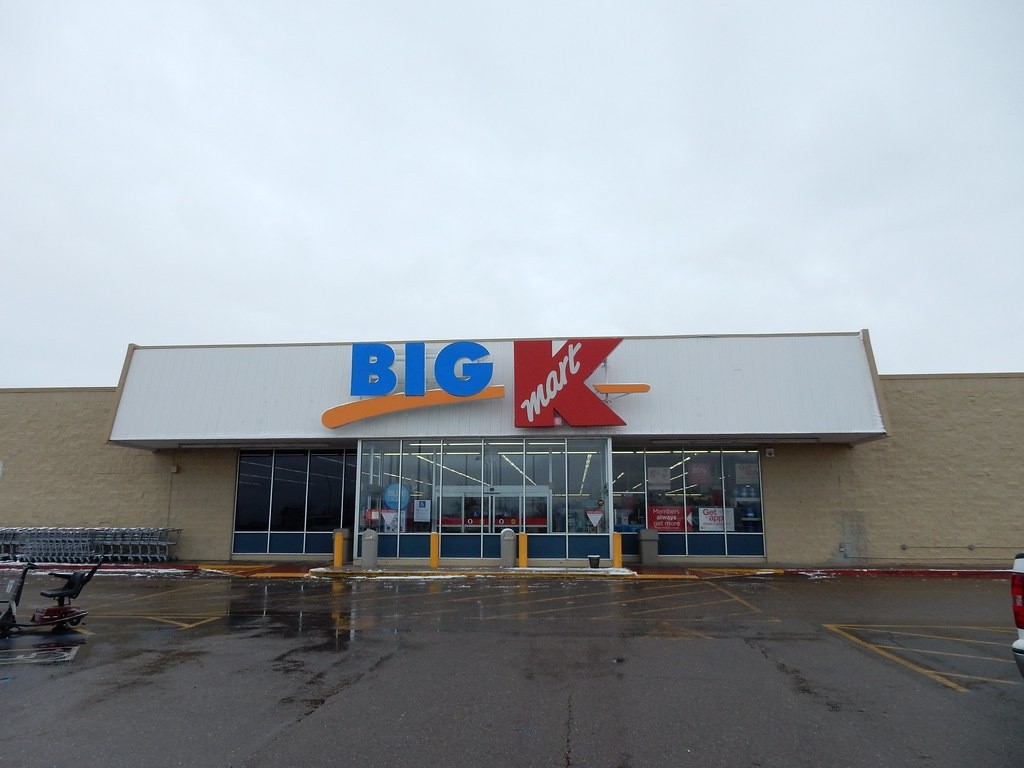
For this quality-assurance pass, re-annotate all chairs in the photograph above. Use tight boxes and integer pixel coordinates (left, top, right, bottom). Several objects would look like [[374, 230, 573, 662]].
[[41, 571, 85, 606]]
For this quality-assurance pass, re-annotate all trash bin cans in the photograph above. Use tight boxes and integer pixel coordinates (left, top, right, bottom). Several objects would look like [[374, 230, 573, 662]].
[[500, 528, 516, 569], [333, 529, 349, 565], [361, 529, 378, 568], [639, 528, 659, 565]]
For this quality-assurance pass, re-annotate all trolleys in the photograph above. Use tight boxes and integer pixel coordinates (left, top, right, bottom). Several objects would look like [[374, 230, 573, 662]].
[[0, 526, 184, 564]]
